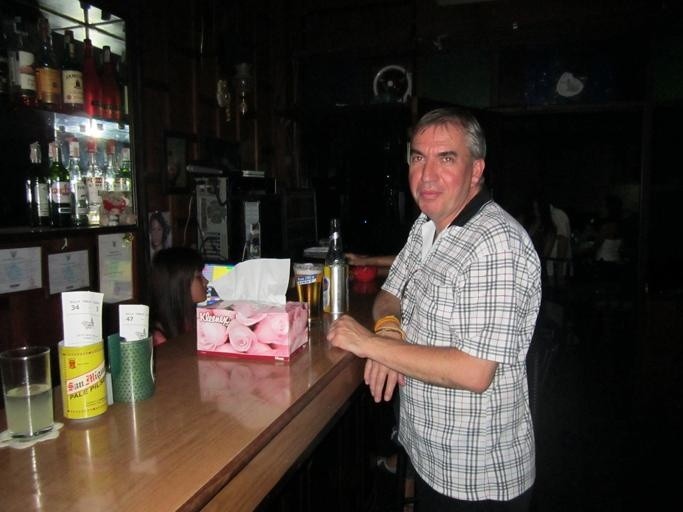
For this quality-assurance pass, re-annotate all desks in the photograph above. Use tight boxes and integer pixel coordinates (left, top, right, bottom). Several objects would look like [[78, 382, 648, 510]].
[[0, 271, 388, 512]]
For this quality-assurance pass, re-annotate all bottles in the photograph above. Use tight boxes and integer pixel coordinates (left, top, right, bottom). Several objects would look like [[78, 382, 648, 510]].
[[101, 46, 121, 122], [66, 138, 88, 225], [35, 18, 62, 112], [9, 17, 40, 110], [82, 139, 104, 225], [101, 140, 119, 226], [59, 30, 84, 116], [48, 141, 73, 227], [26, 141, 51, 228], [119, 49, 129, 124], [83, 39, 103, 119], [323, 219, 349, 312], [117, 142, 133, 225]]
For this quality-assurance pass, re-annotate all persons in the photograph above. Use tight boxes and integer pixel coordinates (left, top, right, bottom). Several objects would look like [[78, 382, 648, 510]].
[[142, 245, 209, 348], [325, 108, 541, 511], [149, 214, 173, 255]]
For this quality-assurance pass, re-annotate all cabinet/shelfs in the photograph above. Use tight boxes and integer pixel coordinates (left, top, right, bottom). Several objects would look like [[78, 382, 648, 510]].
[[0, 3, 149, 403]]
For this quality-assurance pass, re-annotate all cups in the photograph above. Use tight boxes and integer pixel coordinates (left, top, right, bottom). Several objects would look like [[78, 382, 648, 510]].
[[1, 345, 54, 438], [109, 335, 154, 404], [57, 340, 108, 419], [293, 265, 323, 320]]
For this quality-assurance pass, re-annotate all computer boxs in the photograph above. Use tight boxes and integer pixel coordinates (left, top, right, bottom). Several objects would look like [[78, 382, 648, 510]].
[[195, 176, 244, 263], [244, 193, 284, 261]]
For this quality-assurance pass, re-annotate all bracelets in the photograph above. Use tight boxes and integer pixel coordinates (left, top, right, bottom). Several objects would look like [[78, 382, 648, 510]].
[[373, 314, 403, 340]]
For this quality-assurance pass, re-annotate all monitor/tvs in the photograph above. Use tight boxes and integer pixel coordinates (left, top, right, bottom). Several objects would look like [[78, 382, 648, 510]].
[[193, 261, 237, 311]]
